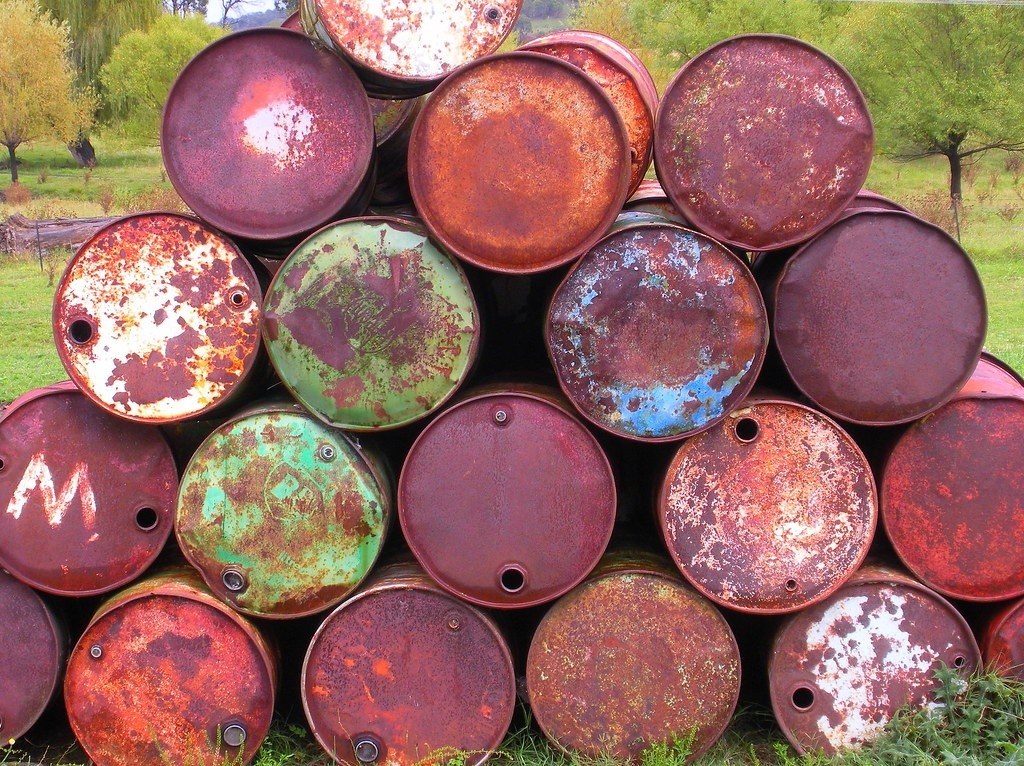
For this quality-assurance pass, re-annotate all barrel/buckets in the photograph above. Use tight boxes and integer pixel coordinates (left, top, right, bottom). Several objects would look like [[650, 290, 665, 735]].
[[0, 0, 1024, 766]]
